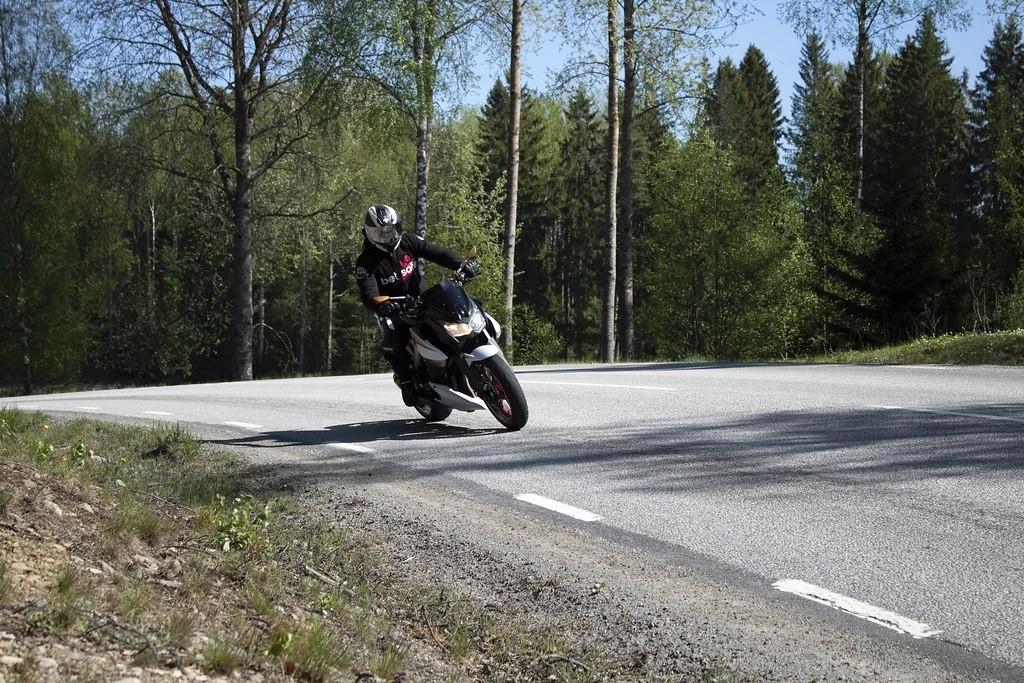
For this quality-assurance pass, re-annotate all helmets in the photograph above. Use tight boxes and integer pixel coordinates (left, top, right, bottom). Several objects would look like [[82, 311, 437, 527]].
[[364, 204, 403, 253]]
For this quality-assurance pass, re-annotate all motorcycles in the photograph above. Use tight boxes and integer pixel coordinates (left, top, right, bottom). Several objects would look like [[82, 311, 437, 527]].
[[370, 245, 529, 431]]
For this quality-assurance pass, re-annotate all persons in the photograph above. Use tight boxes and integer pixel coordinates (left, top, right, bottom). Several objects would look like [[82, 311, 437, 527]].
[[355, 204, 479, 407]]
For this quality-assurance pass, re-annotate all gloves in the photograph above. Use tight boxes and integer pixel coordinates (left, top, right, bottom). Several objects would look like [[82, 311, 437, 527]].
[[377, 301, 397, 319], [460, 261, 481, 278]]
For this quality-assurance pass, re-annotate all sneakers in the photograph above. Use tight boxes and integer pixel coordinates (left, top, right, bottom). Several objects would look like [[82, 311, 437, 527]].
[[399, 378, 419, 407]]
